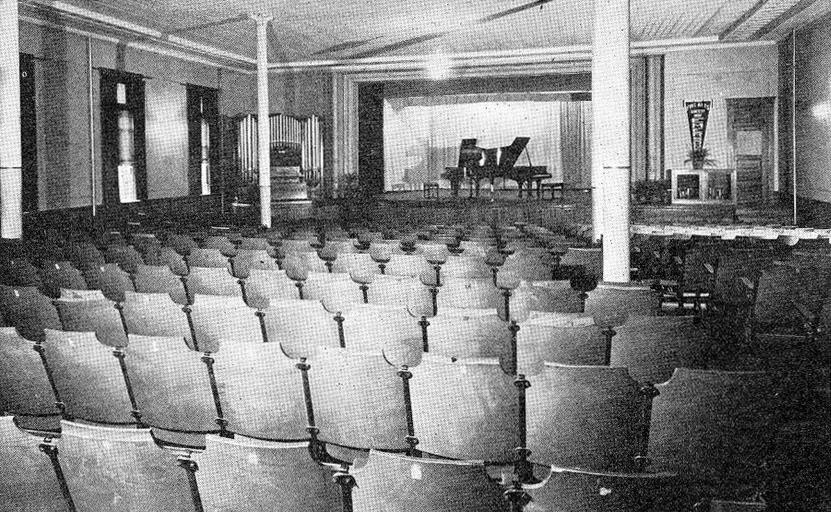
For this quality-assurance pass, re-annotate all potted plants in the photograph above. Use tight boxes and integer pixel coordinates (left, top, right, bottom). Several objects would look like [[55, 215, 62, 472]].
[[684, 145, 719, 170]]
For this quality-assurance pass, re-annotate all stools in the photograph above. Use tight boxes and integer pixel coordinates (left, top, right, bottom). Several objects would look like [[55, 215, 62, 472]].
[[423, 182, 438, 198], [541, 183, 563, 199]]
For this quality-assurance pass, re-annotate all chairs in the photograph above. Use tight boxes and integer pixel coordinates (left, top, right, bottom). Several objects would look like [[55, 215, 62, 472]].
[[0, 217, 830, 510]]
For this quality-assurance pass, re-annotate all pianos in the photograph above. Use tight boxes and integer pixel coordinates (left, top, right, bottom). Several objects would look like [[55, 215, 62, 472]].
[[440, 137, 551, 199]]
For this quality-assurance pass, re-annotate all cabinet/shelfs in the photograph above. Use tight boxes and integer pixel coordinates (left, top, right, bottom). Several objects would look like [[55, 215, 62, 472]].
[[670, 169, 737, 206]]
